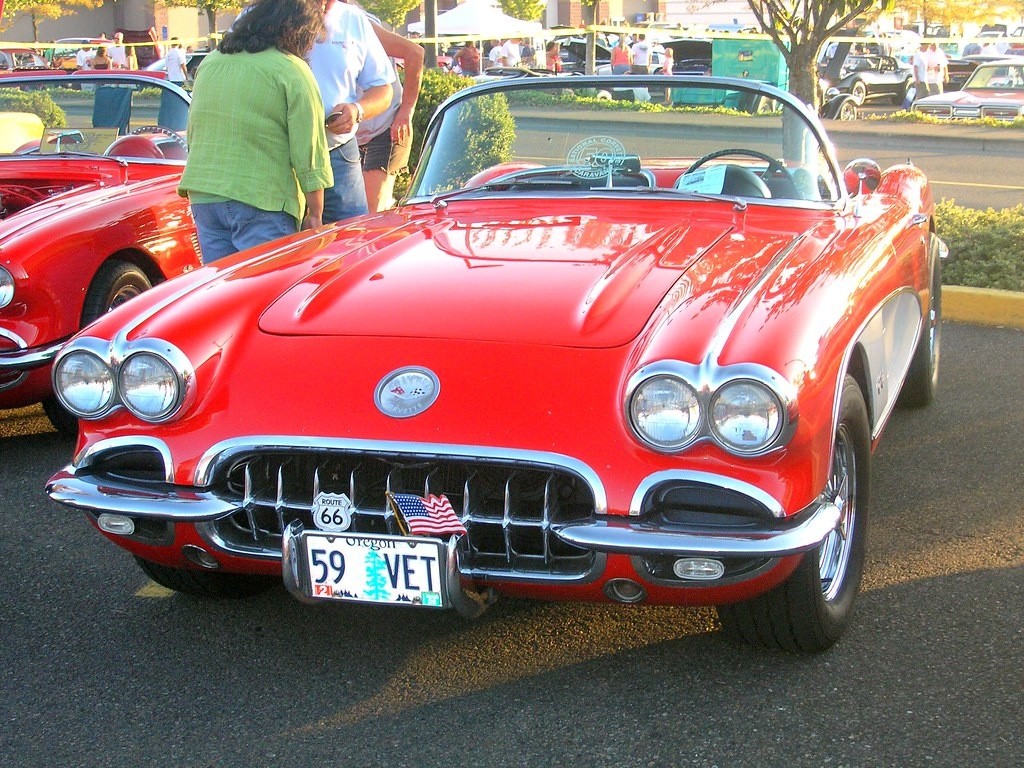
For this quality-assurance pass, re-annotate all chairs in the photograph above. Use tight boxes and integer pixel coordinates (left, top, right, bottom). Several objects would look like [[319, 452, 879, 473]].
[[158, 89, 192, 132], [482, 166, 656, 188], [150, 136, 189, 160], [672, 166, 802, 200], [92, 87, 133, 135]]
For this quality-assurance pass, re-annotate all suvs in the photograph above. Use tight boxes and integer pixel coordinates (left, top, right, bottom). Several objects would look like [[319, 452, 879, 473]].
[[49, 37, 113, 66]]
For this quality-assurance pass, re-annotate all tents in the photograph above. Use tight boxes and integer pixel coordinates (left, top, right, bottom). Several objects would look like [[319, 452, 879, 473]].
[[407, 0, 541, 76]]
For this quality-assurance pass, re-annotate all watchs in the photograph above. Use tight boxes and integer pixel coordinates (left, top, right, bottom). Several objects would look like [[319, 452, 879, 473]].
[[354, 102, 366, 123]]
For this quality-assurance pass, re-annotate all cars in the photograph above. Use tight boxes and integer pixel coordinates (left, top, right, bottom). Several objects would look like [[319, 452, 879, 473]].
[[815, 55, 916, 108], [44, 73, 950, 657], [560, 41, 613, 75], [0, 69, 205, 435], [910, 59, 1023, 121]]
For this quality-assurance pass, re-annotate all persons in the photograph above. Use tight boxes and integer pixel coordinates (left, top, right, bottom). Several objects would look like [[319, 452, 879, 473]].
[[817, 36, 1000, 102], [410, 24, 762, 109], [0, 1, 425, 268]]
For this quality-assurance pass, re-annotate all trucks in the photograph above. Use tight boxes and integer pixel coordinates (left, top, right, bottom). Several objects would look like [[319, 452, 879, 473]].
[[654, 39, 789, 111]]
